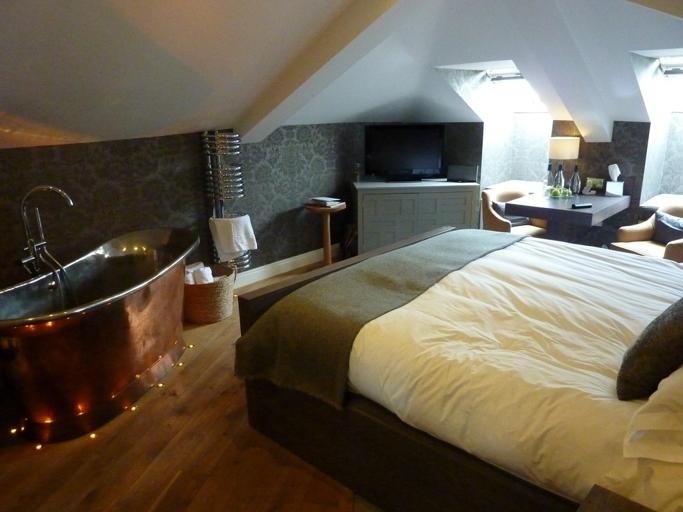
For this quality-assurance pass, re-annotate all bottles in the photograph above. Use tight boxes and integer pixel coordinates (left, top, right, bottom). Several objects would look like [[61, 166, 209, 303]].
[[554, 163, 565, 194], [541, 164, 555, 198], [568, 164, 581, 194]]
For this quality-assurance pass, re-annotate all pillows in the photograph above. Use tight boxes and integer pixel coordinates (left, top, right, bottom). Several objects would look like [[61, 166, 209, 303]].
[[617, 299, 683, 401], [653, 211, 683, 244]]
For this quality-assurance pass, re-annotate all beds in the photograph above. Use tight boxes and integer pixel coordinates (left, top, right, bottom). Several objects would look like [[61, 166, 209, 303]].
[[235, 225, 683, 512]]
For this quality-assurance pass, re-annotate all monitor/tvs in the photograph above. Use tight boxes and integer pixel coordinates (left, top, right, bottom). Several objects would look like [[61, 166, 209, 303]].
[[362, 121, 448, 182]]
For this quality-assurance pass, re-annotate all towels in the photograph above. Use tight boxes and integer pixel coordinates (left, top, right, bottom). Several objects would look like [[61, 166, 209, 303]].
[[184, 261, 213, 284], [209, 215, 258, 263]]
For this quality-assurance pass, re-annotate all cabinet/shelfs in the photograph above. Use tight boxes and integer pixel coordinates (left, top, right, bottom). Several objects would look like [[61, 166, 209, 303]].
[[353, 182, 480, 255]]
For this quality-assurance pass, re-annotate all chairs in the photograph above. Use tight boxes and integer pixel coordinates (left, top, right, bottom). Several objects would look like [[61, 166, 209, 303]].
[[610, 204, 683, 262], [482, 187, 547, 239]]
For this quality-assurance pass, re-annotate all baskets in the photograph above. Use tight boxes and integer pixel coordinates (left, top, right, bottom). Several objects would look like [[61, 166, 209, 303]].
[[184, 264, 235, 325]]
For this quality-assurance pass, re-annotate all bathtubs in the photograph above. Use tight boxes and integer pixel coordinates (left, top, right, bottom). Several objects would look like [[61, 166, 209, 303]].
[[0, 225, 203, 446]]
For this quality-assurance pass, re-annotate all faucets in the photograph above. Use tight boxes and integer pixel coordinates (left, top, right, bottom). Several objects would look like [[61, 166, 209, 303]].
[[19, 179, 75, 253]]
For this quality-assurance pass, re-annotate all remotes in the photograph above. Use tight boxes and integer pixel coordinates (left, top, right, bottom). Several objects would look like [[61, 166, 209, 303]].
[[572, 203, 593, 209]]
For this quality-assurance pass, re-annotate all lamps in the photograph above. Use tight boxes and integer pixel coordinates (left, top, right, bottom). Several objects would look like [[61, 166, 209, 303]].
[[549, 137, 580, 160]]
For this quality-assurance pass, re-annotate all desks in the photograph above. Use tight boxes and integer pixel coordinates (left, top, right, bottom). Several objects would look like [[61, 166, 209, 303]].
[[506, 195, 630, 243], [306, 203, 346, 264]]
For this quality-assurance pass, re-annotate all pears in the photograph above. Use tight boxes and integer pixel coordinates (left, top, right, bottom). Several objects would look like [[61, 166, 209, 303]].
[[550, 188, 560, 196]]
